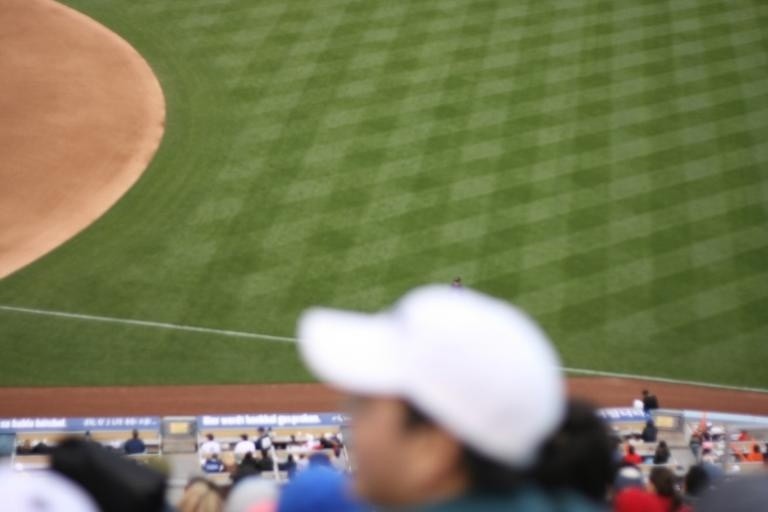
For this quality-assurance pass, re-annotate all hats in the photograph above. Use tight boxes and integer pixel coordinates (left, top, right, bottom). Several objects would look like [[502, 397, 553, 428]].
[[298, 283, 565, 469]]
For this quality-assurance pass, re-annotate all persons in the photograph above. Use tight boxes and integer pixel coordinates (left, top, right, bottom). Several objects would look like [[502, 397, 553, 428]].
[[295, 283, 617, 511], [2, 389, 767, 512]]
